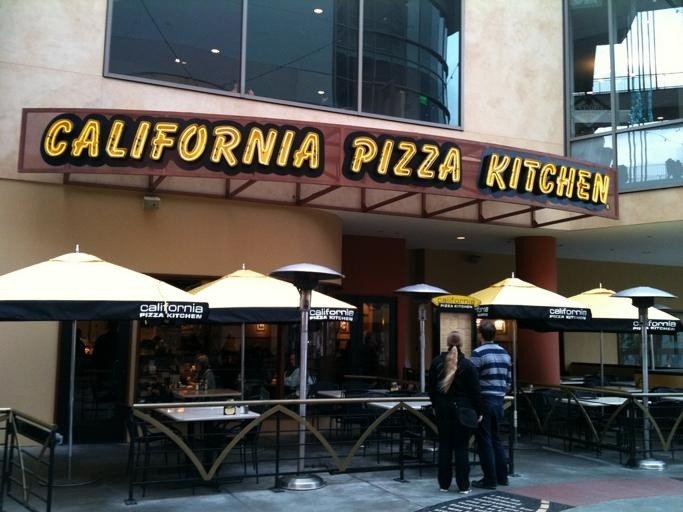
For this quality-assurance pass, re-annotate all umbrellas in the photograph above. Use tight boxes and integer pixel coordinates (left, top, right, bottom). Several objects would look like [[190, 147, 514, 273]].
[[466, 272, 593, 442], [567, 281, 682, 417], [186, 262, 362, 400], [1, 242, 210, 478]]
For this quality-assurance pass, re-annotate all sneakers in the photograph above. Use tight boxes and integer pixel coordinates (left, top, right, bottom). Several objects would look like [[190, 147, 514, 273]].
[[440, 488, 449, 492], [471, 479, 497, 490], [497, 478, 509, 486], [458, 481, 472, 494]]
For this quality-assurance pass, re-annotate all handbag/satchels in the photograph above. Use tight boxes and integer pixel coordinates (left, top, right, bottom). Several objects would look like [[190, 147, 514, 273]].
[[455, 406, 480, 429]]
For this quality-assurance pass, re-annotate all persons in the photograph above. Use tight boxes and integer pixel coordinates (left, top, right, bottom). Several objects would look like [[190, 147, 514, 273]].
[[427, 330, 484, 495], [192, 353, 217, 389], [469, 318, 513, 491], [281, 351, 314, 398]]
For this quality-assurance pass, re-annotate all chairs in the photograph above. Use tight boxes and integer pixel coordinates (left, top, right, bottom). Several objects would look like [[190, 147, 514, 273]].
[[504, 360, 683, 470], [112, 360, 439, 499]]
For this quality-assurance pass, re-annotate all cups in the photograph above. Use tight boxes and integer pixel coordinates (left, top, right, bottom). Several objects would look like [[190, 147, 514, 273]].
[[240, 405, 248, 414]]
